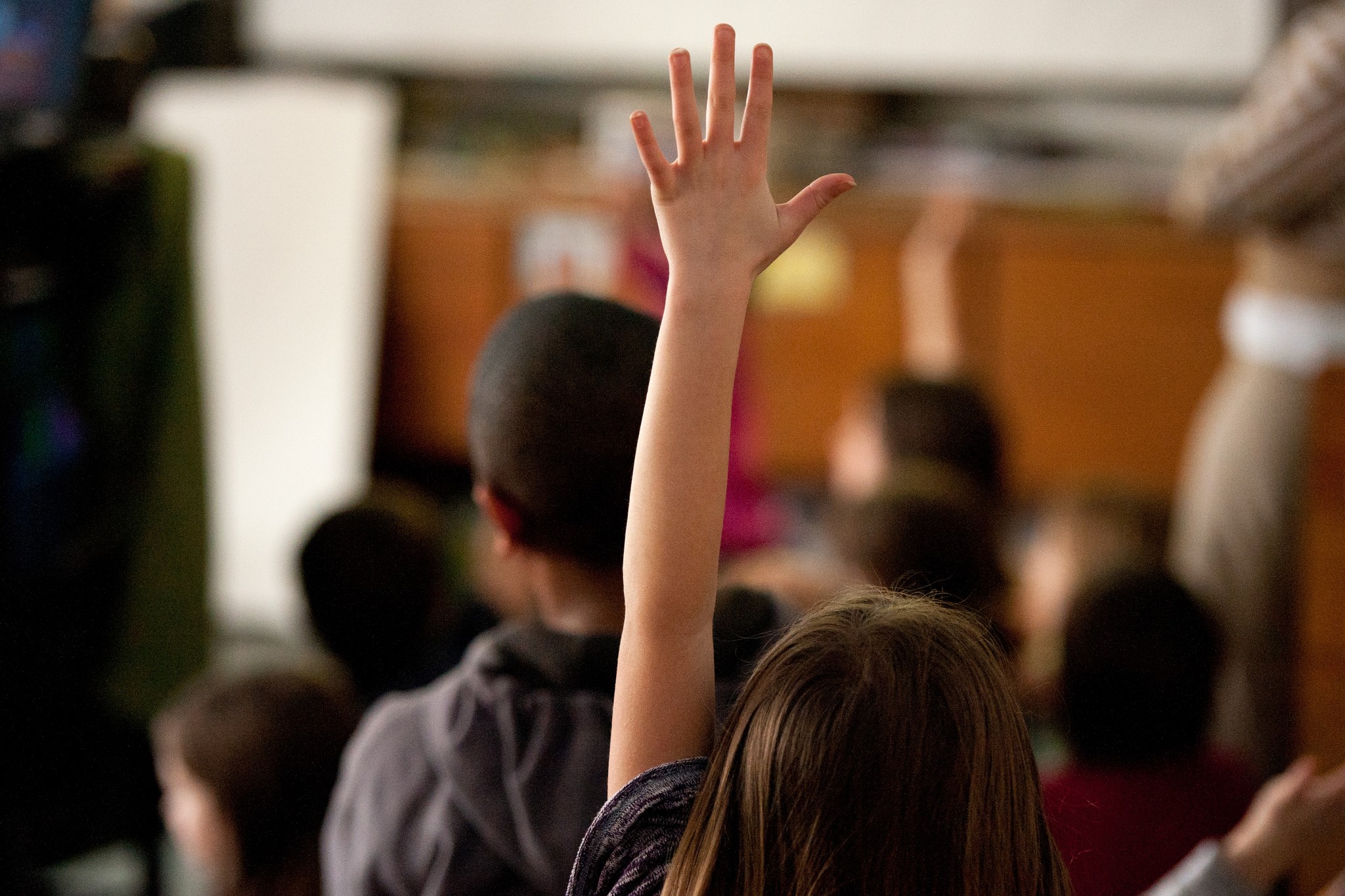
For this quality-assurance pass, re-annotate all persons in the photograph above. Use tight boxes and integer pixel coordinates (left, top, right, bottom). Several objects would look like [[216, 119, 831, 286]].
[[565, 24, 1063, 896], [1142, 755, 1345, 896], [825, 377, 1153, 686], [151, 659, 361, 896], [1031, 565, 1276, 896], [292, 502, 470, 707], [320, 287, 778, 896]]
